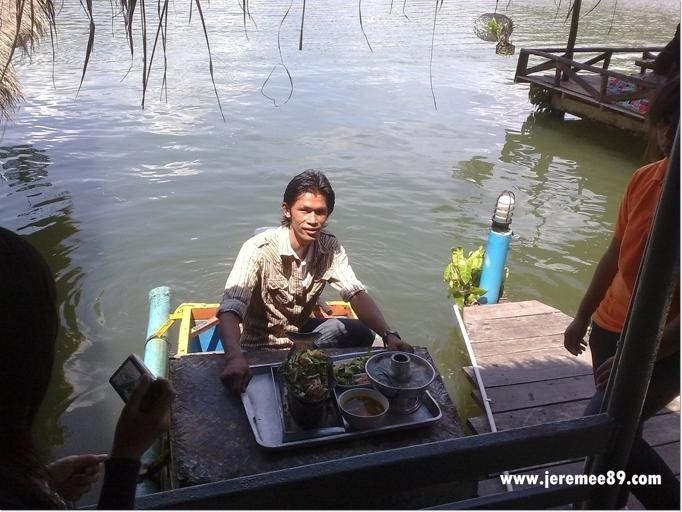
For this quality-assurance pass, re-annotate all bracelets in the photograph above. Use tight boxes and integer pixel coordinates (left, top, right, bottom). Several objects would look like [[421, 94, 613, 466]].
[[382, 330, 401, 348]]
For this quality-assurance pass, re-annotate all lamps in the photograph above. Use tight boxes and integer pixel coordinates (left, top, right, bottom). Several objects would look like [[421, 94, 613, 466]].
[[491, 189, 516, 233]]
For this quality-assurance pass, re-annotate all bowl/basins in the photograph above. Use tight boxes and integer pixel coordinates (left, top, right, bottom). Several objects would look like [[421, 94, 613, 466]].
[[338, 388, 388, 427]]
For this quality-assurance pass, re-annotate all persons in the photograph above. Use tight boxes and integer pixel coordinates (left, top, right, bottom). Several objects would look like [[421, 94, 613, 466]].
[[220, 169, 414, 397], [653, 23, 680, 74], [0, 227, 177, 510], [563, 72, 680, 427]]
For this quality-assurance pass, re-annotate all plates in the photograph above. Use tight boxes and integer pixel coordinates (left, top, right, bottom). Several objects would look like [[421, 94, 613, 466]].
[[333, 357, 369, 388], [271, 366, 345, 437]]
